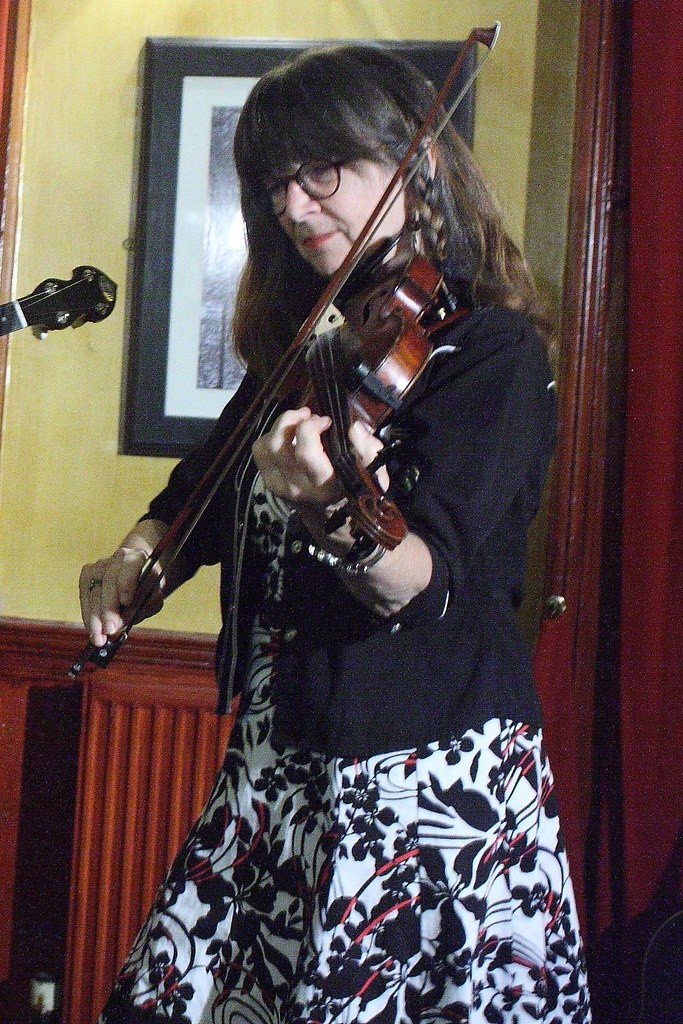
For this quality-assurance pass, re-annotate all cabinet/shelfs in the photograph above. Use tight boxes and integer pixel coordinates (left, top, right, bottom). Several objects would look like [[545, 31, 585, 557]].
[[0, 620, 243, 1024]]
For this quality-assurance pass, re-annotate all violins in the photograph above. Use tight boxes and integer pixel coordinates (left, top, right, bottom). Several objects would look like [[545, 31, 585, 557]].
[[263, 251, 446, 550]]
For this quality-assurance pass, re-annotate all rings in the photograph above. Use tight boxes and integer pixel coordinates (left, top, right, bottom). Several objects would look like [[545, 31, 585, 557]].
[[87, 579, 101, 591]]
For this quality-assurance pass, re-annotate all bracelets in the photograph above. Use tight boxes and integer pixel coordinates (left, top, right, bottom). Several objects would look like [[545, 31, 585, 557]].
[[333, 534, 386, 571]]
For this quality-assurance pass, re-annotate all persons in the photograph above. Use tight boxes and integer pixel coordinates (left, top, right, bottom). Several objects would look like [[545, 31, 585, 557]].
[[80, 45, 592, 1024]]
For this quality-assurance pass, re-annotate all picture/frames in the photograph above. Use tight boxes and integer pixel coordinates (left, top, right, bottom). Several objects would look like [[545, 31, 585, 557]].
[[123, 37, 475, 459]]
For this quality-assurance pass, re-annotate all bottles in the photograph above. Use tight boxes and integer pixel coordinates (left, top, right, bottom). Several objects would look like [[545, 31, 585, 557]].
[[28, 972, 58, 1024]]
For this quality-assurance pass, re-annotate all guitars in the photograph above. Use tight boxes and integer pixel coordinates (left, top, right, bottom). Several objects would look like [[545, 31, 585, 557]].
[[0, 266, 123, 339]]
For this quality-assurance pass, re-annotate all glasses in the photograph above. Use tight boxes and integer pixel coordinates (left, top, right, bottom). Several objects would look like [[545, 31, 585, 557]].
[[259, 139, 388, 216]]
